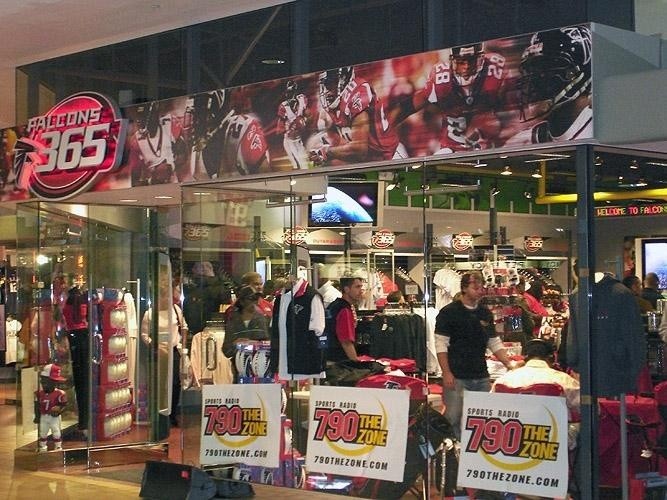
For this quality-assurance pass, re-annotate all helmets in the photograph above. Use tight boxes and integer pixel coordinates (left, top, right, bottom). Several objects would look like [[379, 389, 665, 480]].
[[448, 43, 493, 86], [181, 88, 235, 147], [516, 26, 594, 125], [136, 102, 162, 141], [316, 65, 355, 114]]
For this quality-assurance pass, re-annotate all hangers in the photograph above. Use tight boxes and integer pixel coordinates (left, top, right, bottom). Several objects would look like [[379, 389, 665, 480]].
[[376, 302, 424, 329]]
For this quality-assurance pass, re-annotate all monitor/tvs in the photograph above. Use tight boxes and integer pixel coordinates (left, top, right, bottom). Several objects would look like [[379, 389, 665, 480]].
[[634, 237, 667, 295], [300, 180, 385, 229], [255, 256, 272, 287]]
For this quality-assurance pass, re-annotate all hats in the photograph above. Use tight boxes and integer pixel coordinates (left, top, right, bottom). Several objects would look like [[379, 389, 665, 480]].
[[233, 286, 265, 308], [40, 363, 68, 382], [192, 260, 216, 278]]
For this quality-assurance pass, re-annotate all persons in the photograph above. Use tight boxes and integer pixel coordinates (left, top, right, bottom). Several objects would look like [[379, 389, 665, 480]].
[[623, 272, 665, 311], [486, 339, 582, 500], [62, 287, 89, 442], [557, 271, 643, 399], [432, 274, 513, 500], [269, 277, 327, 380], [0, 126, 35, 203], [32, 365, 69, 452], [140, 260, 278, 429], [321, 277, 363, 369]]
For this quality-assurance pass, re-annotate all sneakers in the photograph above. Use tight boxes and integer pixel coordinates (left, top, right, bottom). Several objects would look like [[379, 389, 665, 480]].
[[53, 443, 63, 452], [34, 439, 48, 454]]
